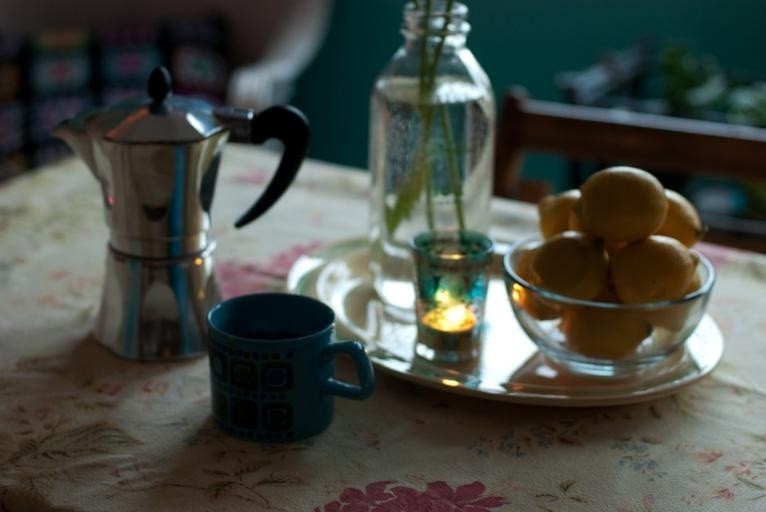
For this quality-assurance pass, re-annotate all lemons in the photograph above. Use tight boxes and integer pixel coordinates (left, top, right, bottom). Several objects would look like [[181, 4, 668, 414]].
[[515, 164, 705, 360]]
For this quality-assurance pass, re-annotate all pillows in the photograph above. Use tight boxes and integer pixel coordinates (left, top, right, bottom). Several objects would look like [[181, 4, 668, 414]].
[[25, 15, 232, 169]]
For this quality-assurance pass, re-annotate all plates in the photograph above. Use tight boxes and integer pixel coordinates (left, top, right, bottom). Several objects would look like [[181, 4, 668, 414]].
[[288, 237, 725, 408]]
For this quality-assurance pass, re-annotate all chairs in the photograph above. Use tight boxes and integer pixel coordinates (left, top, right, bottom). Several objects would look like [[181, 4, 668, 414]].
[[494, 85, 766, 255], [0, 0, 332, 181]]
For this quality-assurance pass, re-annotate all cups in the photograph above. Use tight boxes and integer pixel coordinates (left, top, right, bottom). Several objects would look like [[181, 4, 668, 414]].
[[410, 231, 495, 366], [205, 292, 376, 444]]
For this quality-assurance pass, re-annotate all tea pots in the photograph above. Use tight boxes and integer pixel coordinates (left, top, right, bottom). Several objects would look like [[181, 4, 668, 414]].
[[51, 66, 310, 362]]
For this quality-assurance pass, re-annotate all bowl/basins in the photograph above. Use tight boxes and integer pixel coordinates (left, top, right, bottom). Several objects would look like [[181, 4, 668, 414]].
[[501, 236, 715, 377]]
